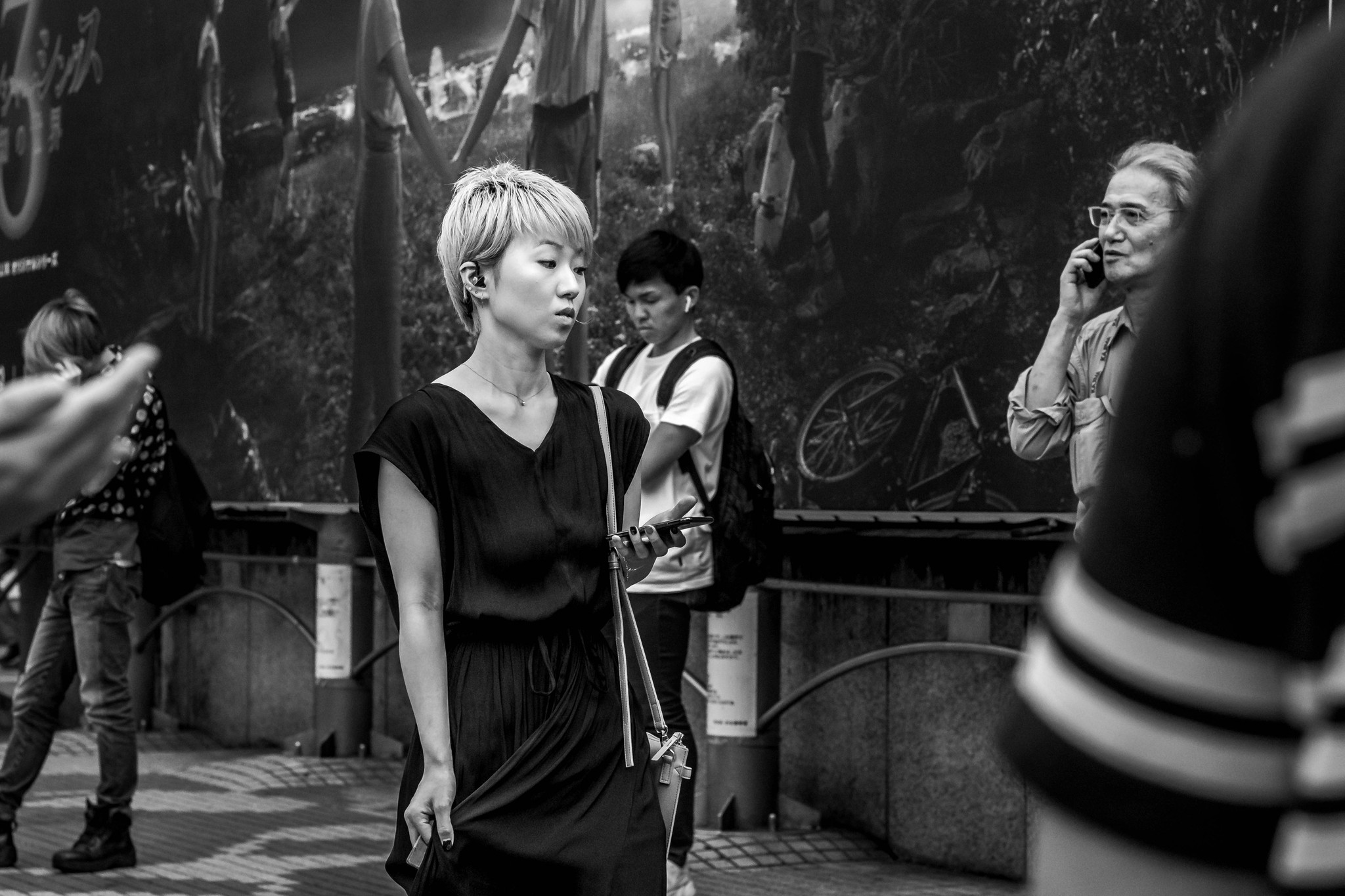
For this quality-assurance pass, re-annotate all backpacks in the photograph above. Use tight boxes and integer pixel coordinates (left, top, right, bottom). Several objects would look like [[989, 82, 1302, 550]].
[[605, 339, 786, 613], [137, 384, 216, 615]]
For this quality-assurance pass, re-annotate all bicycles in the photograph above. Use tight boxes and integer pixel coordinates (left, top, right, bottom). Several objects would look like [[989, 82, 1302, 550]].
[[793, 272, 1018, 514]]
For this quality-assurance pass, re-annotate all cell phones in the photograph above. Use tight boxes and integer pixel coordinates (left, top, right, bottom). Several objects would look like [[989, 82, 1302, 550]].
[[604, 516, 714, 541], [1083, 243, 1106, 288]]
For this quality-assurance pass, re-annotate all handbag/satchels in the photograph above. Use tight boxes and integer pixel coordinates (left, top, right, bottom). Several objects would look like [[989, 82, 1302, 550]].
[[646, 731, 692, 860]]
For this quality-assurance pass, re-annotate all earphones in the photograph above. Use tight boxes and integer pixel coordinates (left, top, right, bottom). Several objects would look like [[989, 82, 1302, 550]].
[[684, 296, 692, 313]]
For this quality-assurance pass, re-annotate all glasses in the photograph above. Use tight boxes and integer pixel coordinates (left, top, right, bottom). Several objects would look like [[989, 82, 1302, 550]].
[[1088, 206, 1178, 230]]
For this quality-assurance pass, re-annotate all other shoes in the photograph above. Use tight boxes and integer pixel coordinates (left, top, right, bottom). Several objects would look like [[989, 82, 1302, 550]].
[[666, 859, 695, 896]]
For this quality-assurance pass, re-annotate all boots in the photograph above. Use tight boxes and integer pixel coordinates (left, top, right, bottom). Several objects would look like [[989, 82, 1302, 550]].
[[51, 798, 137, 872], [0, 819, 18, 866]]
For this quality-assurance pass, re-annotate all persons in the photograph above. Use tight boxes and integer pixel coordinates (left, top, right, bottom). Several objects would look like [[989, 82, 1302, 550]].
[[989, 27, 1345, 896], [0, 287, 219, 875], [352, 163, 683, 895], [586, 223, 733, 896]]
